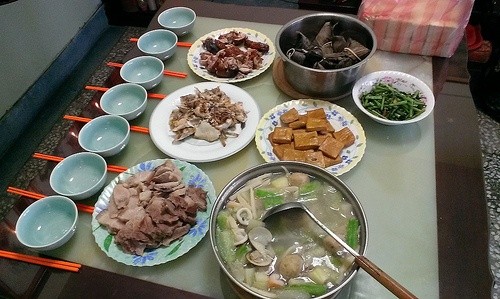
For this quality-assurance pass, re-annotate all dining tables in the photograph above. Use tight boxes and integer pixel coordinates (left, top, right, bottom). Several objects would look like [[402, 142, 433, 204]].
[[0, 0, 496, 299]]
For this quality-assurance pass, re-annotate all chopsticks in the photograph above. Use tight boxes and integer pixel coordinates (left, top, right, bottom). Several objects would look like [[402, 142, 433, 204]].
[[7, 186, 94, 213], [0, 250, 81, 273], [33, 153, 129, 173], [107, 62, 187, 78], [129, 37, 192, 47], [85, 85, 167, 99], [63, 115, 149, 133]]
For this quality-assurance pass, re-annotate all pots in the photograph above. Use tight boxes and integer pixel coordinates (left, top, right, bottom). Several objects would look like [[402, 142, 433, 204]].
[[209, 162, 369, 299]]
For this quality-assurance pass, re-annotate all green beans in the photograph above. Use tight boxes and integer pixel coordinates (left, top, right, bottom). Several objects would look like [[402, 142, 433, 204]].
[[217, 179, 359, 296]]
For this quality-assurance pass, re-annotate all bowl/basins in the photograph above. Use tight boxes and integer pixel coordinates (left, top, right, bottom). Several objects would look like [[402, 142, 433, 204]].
[[157, 7, 196, 36], [100, 83, 147, 121], [50, 152, 107, 200], [78, 115, 130, 158], [137, 29, 178, 61], [352, 70, 435, 125], [275, 13, 377, 98], [15, 195, 78, 251], [120, 55, 164, 90]]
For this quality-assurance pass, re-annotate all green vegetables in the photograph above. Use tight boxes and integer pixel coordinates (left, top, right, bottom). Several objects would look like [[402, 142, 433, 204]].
[[362, 83, 426, 122]]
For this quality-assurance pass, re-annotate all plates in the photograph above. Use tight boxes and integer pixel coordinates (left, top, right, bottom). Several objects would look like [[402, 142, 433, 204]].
[[186, 27, 275, 84], [148, 82, 260, 163], [91, 158, 216, 267], [255, 99, 367, 177]]
